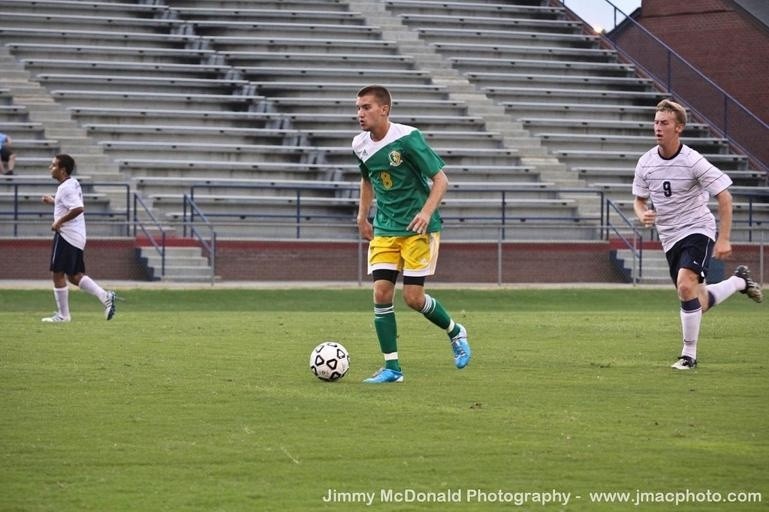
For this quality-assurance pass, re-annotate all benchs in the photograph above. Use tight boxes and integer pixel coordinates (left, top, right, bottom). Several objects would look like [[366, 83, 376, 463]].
[[0, 1, 769, 251]]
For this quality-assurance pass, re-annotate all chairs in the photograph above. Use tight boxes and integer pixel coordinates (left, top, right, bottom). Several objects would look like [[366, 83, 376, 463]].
[[670, 356, 697, 370], [733, 265, 763, 303], [451, 321, 472, 369], [41, 314, 71, 322], [361, 364, 404, 383], [103, 290, 117, 321]]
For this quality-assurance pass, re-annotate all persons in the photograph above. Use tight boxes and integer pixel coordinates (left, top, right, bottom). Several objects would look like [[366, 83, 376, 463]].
[[631, 99, 763, 372], [40, 154, 116, 323], [0, 133, 18, 182], [350, 85, 474, 383]]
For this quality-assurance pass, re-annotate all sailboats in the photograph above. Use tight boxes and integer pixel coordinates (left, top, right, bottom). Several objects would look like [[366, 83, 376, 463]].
[[310, 342, 350, 382]]
